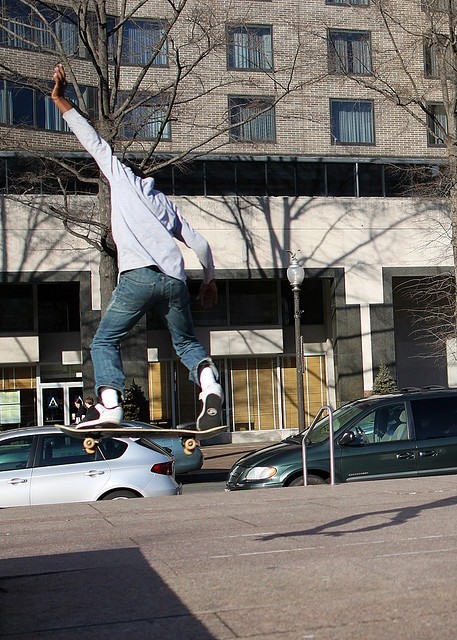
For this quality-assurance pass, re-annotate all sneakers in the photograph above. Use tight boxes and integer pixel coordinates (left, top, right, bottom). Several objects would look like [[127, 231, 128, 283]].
[[196, 384, 224, 430], [76, 402, 123, 428]]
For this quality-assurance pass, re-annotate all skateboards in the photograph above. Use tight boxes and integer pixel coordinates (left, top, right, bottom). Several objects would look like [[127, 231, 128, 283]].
[[54, 423, 229, 456]]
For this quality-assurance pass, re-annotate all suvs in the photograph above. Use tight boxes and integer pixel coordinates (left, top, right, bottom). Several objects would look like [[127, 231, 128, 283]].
[[224, 385, 457, 491], [0, 426, 183, 509]]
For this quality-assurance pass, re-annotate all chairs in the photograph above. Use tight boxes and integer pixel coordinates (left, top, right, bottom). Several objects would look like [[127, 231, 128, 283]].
[[381, 409, 401, 442], [388, 410, 408, 441]]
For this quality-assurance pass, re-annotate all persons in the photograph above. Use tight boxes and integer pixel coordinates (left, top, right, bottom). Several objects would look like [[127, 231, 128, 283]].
[[85, 396, 94, 408], [50, 62, 225, 432], [75, 398, 86, 423]]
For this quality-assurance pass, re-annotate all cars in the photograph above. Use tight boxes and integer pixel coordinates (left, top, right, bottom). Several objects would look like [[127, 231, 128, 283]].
[[121, 420, 203, 475]]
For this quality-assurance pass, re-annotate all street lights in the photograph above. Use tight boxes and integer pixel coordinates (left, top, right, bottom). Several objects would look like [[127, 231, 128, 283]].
[[285, 248, 306, 434]]
